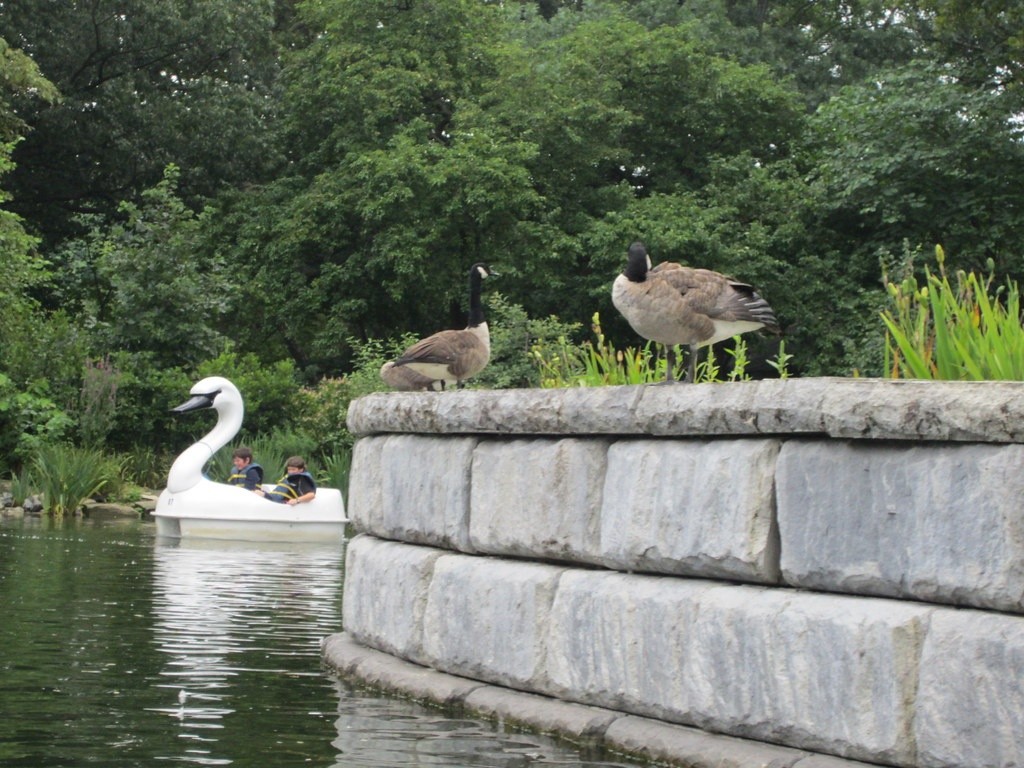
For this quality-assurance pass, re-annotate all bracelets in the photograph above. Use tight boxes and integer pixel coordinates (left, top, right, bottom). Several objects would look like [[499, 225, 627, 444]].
[[296, 498, 300, 504]]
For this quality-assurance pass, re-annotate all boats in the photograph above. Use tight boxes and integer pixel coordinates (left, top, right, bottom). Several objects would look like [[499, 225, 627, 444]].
[[150, 376, 352, 543]]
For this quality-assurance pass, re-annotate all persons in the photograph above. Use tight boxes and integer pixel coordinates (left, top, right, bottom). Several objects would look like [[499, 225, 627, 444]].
[[253, 456, 316, 506], [227, 448, 264, 492]]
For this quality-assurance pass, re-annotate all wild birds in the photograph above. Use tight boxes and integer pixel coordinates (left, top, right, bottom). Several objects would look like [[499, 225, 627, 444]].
[[610, 240, 780, 388], [377, 262, 492, 393]]
